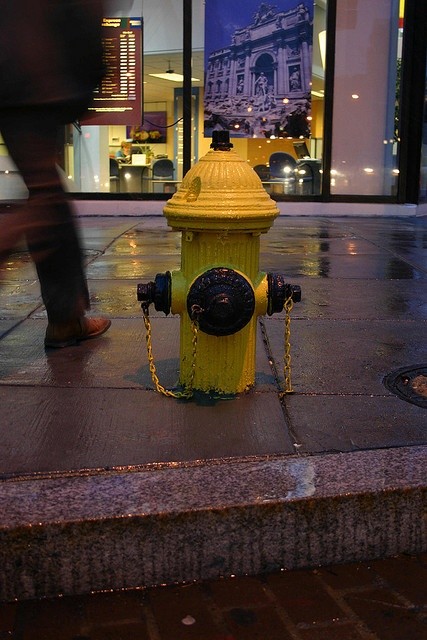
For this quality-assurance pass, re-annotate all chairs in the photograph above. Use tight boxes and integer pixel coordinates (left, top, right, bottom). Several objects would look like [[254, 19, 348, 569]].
[[253, 163, 268, 182], [140, 158, 174, 194], [110, 158, 121, 193], [268, 151, 297, 195]]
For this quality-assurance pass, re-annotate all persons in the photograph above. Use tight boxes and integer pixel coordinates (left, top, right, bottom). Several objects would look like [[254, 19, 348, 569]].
[[0, 1, 113, 348], [289, 67, 300, 92], [254, 71, 269, 98], [115, 141, 132, 164], [237, 76, 244, 94]]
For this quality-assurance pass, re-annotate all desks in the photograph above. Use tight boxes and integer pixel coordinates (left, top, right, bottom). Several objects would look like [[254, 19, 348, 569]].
[[118, 163, 150, 194]]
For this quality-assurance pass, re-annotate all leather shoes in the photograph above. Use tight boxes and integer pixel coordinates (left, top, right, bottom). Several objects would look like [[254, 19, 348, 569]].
[[44, 317, 111, 347]]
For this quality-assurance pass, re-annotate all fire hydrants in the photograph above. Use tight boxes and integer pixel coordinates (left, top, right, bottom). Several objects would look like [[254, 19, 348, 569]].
[[137, 130, 302, 399]]
